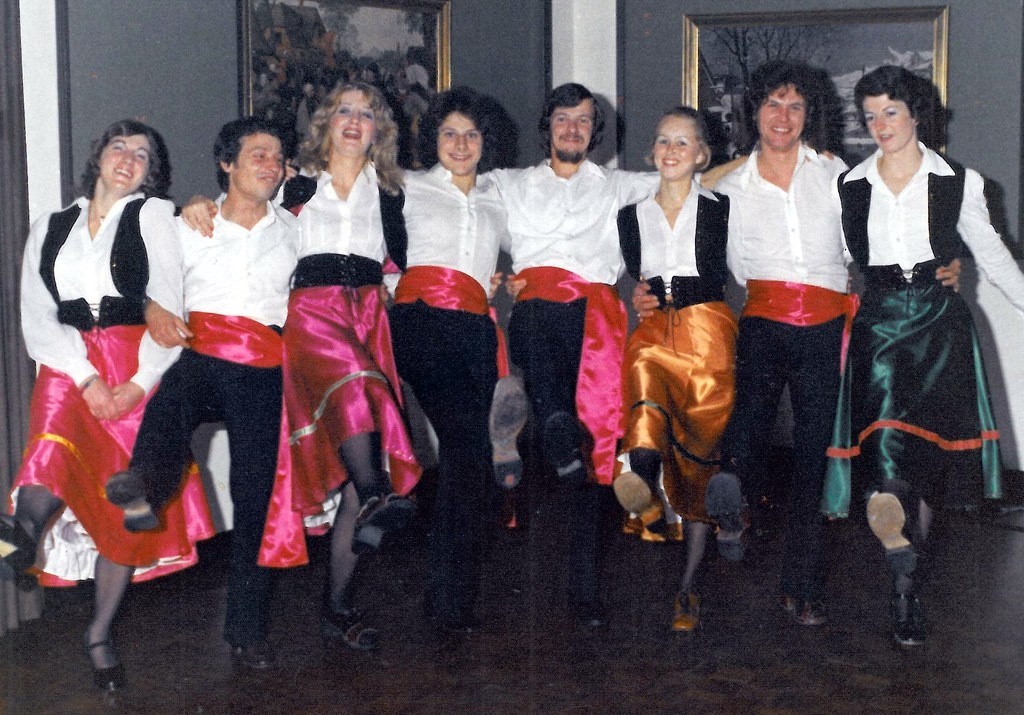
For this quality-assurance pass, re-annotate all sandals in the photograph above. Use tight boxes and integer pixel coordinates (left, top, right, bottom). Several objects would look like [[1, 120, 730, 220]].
[[322, 614, 384, 649], [356, 492, 416, 528]]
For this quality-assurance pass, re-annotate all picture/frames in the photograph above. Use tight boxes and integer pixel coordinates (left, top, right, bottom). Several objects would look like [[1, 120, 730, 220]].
[[236, 0, 451, 171], [681, 5, 950, 162]]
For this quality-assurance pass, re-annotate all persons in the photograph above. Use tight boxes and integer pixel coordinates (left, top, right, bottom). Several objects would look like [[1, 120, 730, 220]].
[[0, 119, 189, 689], [282, 87, 520, 638], [488, 55, 1024, 648], [182, 78, 496, 649], [104, 118, 390, 671]]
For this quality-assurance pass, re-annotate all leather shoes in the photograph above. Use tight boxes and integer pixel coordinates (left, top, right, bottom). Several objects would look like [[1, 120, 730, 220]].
[[866, 490, 910, 550], [84, 627, 126, 690], [0, 520, 38, 571], [891, 593, 927, 645]]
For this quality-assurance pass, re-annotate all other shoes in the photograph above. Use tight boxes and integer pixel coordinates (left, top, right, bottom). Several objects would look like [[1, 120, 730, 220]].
[[570, 597, 605, 628], [422, 607, 480, 632], [488, 374, 529, 488], [704, 472, 755, 559], [224, 631, 274, 667], [105, 473, 160, 534], [672, 591, 702, 631], [543, 412, 582, 487], [773, 592, 828, 625], [614, 469, 666, 534]]
